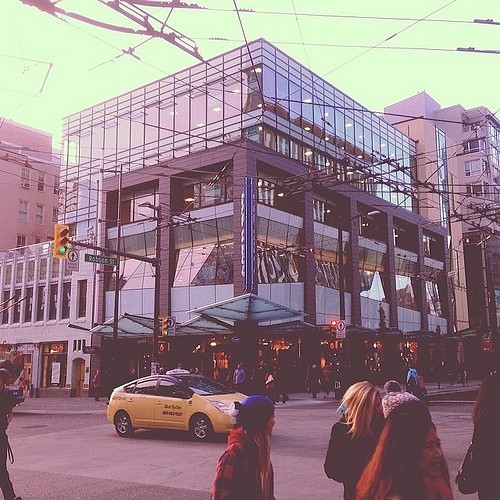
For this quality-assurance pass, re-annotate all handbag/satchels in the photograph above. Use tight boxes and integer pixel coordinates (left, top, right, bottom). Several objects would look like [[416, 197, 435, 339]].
[[406, 371, 416, 393]]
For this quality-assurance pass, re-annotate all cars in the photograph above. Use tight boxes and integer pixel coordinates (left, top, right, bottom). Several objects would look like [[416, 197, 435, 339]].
[[106, 367, 250, 441]]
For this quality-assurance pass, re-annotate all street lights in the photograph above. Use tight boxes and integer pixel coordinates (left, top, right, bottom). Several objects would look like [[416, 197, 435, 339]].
[[335, 211, 381, 323], [138, 201, 162, 369]]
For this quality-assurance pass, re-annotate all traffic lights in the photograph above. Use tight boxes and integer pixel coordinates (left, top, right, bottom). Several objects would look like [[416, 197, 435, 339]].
[[330, 319, 337, 338], [54, 223, 70, 260], [157, 317, 170, 338]]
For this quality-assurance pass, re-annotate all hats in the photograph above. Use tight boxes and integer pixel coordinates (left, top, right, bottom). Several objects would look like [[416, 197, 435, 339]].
[[232, 395, 275, 427], [381, 380, 431, 419]]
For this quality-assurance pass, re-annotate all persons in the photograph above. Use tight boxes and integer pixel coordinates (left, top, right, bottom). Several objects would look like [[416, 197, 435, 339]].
[[0, 361, 500, 500], [212, 395, 279, 500]]
